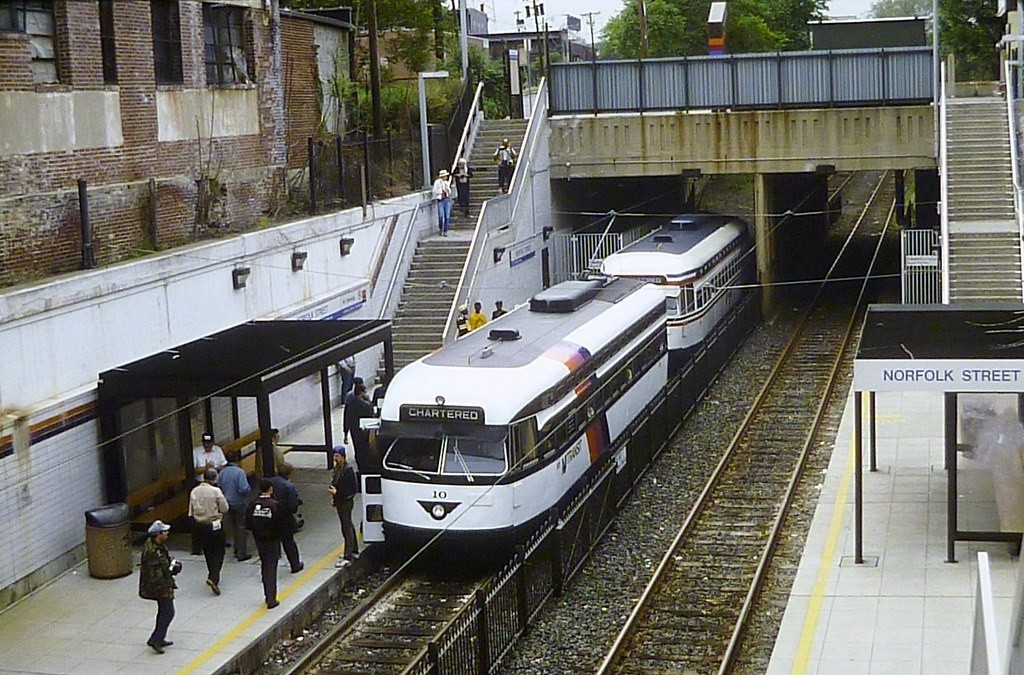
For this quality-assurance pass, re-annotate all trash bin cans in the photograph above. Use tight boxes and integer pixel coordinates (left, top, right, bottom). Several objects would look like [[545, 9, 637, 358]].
[[85, 503, 133, 579], [960, 405, 999, 460], [522, 86, 538, 119]]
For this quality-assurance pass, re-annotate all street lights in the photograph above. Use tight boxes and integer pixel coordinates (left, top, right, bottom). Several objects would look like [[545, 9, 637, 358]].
[[524, 0, 544, 77], [418, 70, 449, 191]]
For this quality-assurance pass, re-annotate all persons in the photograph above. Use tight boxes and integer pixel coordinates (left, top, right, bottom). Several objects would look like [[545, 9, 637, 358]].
[[254, 428, 284, 481], [345, 377, 370, 406], [467, 301, 488, 331], [193, 432, 231, 547], [371, 374, 387, 416], [432, 170, 451, 237], [246, 479, 295, 609], [456, 304, 470, 336], [217, 449, 253, 562], [269, 461, 304, 573], [492, 299, 508, 321], [492, 138, 517, 194], [139, 519, 183, 654], [452, 158, 473, 219], [188, 467, 229, 596], [327, 446, 359, 568], [343, 385, 373, 478], [336, 356, 357, 408]]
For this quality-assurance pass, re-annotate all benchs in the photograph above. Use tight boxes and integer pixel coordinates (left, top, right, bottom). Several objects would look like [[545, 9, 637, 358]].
[[127, 433, 292, 524]]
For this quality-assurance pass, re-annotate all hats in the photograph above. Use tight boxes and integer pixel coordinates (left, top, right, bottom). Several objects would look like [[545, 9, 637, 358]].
[[457, 305, 467, 313], [333, 446, 346, 456], [147, 520, 171, 536], [202, 433, 214, 441], [438, 170, 449, 177], [204, 468, 217, 480]]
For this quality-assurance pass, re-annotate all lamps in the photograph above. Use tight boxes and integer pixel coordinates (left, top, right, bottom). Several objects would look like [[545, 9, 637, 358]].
[[340, 238, 354, 255], [493, 247, 505, 261], [292, 251, 308, 272], [232, 268, 249, 289], [544, 226, 553, 239]]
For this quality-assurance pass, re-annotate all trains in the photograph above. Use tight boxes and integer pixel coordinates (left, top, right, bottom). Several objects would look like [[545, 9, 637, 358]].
[[360, 211, 755, 561]]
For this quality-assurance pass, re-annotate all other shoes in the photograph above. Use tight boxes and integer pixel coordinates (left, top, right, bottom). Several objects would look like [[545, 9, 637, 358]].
[[161, 640, 173, 647], [147, 639, 166, 654], [439, 231, 443, 236], [335, 559, 353, 567], [291, 562, 303, 573], [339, 552, 359, 559], [238, 554, 252, 561], [444, 233, 447, 237], [268, 599, 279, 609], [207, 579, 220, 595]]
